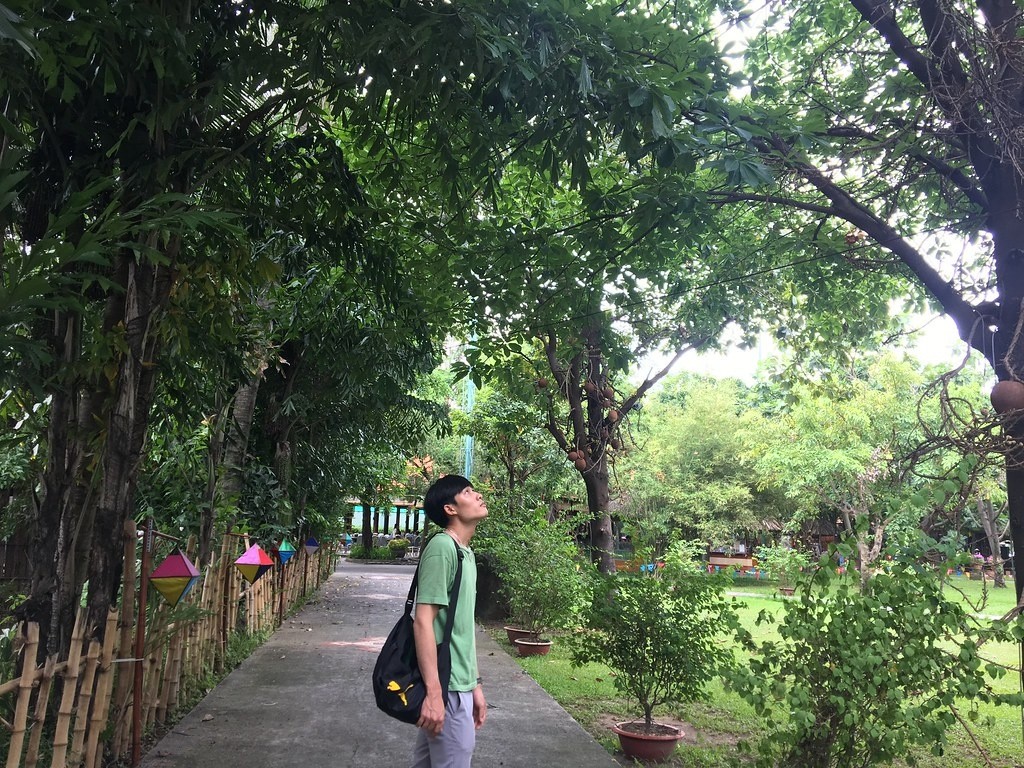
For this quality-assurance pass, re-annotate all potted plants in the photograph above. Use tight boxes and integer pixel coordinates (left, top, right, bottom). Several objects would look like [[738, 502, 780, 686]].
[[555, 562, 758, 764], [388, 538, 411, 558], [752, 539, 812, 596], [469, 492, 622, 656]]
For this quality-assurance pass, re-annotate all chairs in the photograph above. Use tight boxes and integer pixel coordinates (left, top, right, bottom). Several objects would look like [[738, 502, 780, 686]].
[[339, 532, 421, 557]]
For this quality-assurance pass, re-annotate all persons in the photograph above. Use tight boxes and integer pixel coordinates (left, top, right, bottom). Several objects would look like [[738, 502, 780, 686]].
[[393, 524, 398, 538], [395, 529, 420, 539], [970, 548, 984, 571], [414, 475, 488, 768]]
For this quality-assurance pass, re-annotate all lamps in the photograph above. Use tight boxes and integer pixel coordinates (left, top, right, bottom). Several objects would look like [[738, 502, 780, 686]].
[[339, 531, 353, 547], [234, 543, 275, 585], [302, 536, 320, 556], [270, 536, 297, 565], [148, 545, 200, 611]]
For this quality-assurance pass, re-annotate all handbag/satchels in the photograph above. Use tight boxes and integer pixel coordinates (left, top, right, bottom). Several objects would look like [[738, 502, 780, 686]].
[[372, 614, 451, 727]]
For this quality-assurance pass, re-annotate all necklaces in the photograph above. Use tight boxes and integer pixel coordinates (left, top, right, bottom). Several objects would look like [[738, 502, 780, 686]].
[[449, 528, 462, 542]]
